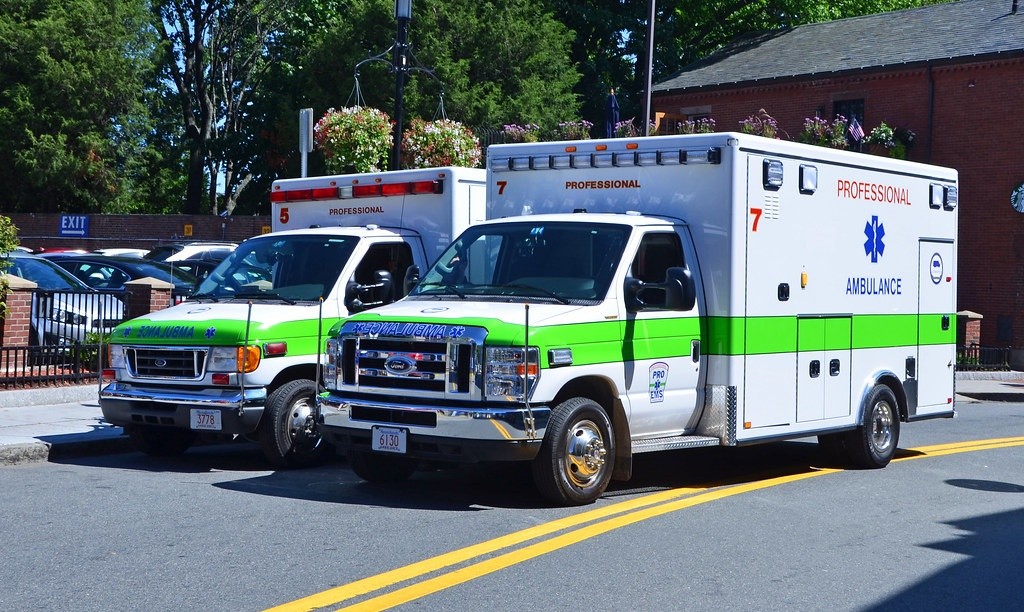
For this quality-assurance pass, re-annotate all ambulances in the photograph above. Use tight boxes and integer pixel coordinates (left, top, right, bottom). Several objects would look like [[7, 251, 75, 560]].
[[309, 131, 959, 506], [97, 166, 486, 471]]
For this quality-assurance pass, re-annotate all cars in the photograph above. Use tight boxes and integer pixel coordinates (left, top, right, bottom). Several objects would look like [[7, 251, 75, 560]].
[[0, 241, 270, 367]]
